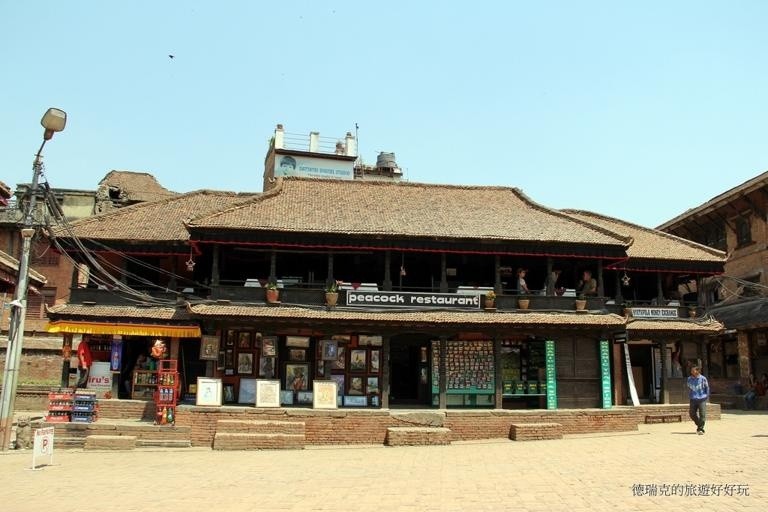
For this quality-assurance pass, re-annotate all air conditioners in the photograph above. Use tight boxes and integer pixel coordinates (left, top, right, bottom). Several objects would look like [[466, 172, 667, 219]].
[[688, 304, 698, 320]]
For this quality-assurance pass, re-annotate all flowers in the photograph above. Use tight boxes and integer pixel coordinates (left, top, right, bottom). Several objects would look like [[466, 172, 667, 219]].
[[486, 290, 496, 300]]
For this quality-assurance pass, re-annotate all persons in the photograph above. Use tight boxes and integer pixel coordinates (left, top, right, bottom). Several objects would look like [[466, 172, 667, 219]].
[[136, 350, 153, 369], [76, 332, 94, 388], [686, 365, 710, 435], [517, 267, 533, 296], [578, 270, 598, 298], [545, 266, 565, 296], [742, 370, 759, 411]]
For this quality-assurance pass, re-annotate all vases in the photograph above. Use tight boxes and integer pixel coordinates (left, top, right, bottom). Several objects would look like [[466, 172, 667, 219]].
[[519, 300, 530, 309], [485, 300, 494, 308]]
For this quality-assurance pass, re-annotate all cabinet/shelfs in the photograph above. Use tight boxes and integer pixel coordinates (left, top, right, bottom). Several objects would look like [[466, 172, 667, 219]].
[[132, 370, 181, 401]]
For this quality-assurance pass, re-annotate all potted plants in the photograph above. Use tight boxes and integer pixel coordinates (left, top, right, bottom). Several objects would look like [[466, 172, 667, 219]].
[[575, 294, 587, 310], [265, 280, 279, 302], [324, 280, 339, 305]]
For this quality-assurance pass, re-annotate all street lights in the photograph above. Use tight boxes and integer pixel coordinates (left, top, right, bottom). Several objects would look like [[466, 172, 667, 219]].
[[0, 108, 67, 452]]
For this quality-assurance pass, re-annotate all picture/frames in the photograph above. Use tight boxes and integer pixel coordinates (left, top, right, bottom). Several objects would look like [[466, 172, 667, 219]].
[[200, 326, 383, 407]]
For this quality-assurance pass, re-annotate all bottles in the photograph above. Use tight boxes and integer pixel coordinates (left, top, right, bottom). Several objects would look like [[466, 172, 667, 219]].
[[157, 406, 173, 424], [160, 373, 174, 385], [138, 373, 158, 384], [48, 387, 97, 417], [159, 388, 174, 402]]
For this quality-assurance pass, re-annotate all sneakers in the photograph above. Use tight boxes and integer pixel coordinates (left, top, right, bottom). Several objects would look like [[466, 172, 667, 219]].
[[696, 426, 705, 435]]
